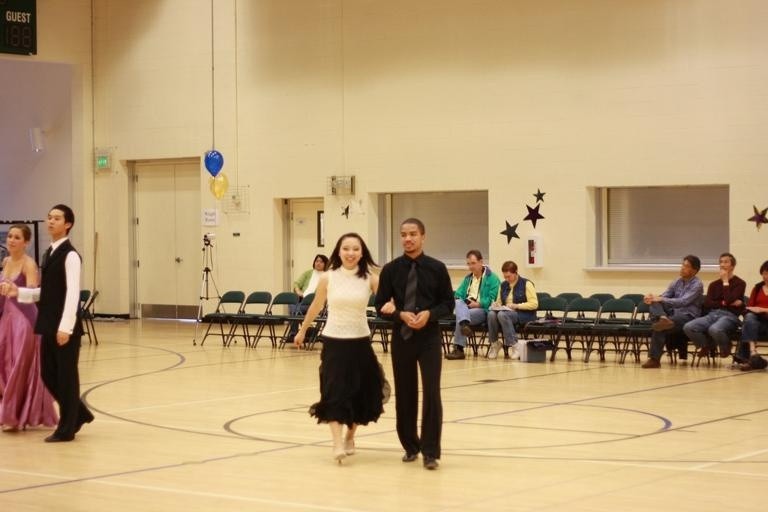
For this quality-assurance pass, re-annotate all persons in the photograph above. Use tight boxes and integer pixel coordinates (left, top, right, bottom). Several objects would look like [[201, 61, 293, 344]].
[[733, 261, 767, 372], [0, 224, 61, 432], [0, 204, 95, 443], [487, 260, 539, 360], [293, 233, 396, 464], [445, 249, 502, 360], [286, 254, 330, 342], [640, 255, 704, 368], [374, 217, 456, 471], [682, 252, 747, 358]]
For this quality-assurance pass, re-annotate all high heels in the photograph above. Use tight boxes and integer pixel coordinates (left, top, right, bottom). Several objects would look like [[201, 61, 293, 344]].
[[334, 439, 355, 465]]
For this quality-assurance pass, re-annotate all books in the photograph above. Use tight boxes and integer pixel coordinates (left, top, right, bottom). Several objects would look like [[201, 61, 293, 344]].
[[488, 304, 514, 311], [528, 319, 562, 326]]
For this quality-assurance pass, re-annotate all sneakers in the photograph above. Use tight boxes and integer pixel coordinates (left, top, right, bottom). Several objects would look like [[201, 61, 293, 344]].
[[445, 324, 521, 360], [642, 318, 760, 370]]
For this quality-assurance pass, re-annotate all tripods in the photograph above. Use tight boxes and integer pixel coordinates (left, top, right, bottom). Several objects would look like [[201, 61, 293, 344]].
[[192, 247, 238, 347]]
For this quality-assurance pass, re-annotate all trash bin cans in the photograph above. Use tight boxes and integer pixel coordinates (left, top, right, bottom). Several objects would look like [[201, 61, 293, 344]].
[[528, 341, 546, 362]]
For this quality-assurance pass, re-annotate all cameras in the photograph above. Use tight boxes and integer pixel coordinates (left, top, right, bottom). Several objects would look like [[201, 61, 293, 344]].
[[465, 298, 476, 305], [204, 230, 213, 246]]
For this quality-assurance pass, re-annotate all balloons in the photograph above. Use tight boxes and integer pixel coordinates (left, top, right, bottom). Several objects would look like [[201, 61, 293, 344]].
[[204, 150, 224, 178], [208, 171, 229, 200]]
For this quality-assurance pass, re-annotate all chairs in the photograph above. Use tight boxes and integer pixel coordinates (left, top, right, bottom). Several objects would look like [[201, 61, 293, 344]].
[[200, 291, 768, 370], [82, 291, 99, 345], [80, 290, 90, 309]]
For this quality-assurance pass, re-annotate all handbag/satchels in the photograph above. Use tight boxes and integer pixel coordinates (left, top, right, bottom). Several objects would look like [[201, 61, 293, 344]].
[[528, 316, 559, 329]]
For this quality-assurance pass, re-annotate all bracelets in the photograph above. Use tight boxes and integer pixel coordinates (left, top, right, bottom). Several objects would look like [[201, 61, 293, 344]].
[[302, 322, 312, 327]]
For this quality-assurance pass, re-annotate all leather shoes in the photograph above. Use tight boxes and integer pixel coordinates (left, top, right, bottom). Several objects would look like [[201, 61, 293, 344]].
[[45, 413, 94, 442], [402, 453, 417, 461], [424, 455, 437, 468]]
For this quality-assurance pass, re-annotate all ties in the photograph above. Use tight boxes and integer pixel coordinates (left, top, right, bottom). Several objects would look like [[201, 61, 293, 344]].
[[399, 262, 418, 341]]
